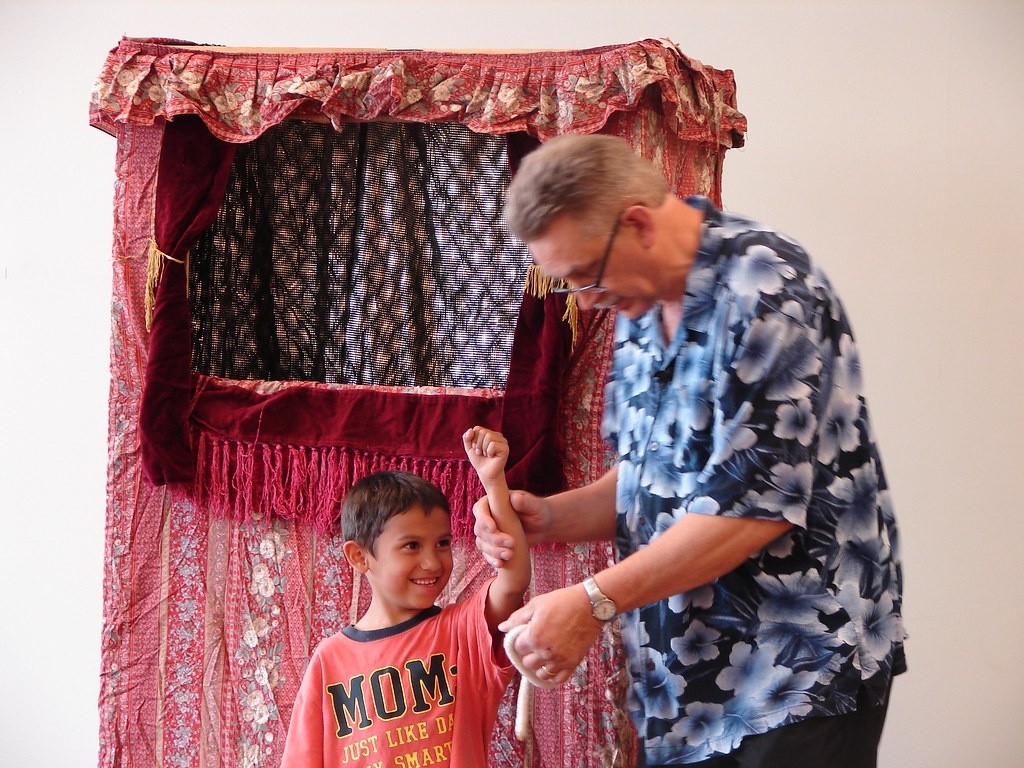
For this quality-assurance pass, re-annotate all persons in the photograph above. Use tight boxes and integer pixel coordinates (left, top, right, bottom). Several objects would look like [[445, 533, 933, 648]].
[[472, 134, 907, 768], [280, 426, 531, 768]]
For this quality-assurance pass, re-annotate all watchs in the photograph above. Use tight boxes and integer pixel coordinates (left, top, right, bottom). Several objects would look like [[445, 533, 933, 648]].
[[583, 575, 618, 623]]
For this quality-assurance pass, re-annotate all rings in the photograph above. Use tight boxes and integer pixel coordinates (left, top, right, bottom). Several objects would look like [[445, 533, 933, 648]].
[[541, 666, 556, 676]]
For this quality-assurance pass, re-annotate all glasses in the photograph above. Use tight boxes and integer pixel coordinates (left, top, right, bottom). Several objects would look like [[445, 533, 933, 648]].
[[553, 202, 649, 294]]
